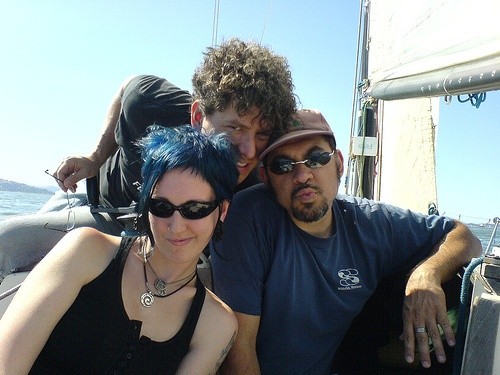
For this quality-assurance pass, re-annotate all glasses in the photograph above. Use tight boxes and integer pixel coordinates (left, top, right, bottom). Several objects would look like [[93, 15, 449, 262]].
[[145, 198, 217, 220], [268, 150, 335, 175]]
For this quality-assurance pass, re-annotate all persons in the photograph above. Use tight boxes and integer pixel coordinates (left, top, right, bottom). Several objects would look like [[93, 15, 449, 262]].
[[210, 107, 483, 375], [1, 39, 300, 283], [0, 126, 239, 375]]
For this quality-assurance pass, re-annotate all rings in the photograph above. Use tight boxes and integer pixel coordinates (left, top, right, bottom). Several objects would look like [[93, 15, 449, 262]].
[[415, 327, 427, 333]]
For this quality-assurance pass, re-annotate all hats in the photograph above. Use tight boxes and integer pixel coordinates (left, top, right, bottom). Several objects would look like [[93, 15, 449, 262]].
[[258, 109, 333, 160]]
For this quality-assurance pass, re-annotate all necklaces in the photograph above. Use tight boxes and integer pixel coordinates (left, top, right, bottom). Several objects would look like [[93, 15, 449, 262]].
[[140, 236, 200, 308], [144, 253, 196, 295]]
